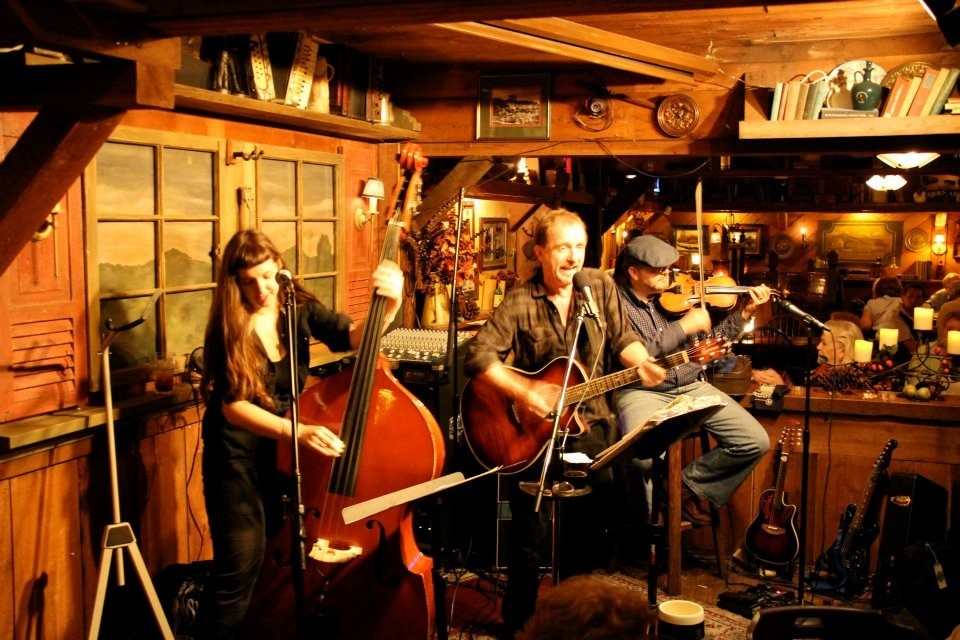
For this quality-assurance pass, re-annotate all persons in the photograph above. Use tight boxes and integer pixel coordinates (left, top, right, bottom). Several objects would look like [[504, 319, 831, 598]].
[[463, 208, 669, 640], [516, 576, 658, 640], [199, 228, 406, 640], [603, 235, 772, 525], [816, 318, 864, 369], [860, 272, 960, 390]]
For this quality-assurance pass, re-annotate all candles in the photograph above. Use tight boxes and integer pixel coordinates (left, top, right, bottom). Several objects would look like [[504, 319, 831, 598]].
[[913, 306, 933, 330], [878, 327, 899, 349], [947, 330, 960, 356], [854, 338, 873, 363]]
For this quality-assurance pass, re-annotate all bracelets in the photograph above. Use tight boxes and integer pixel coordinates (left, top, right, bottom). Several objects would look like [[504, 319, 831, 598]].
[[383, 313, 395, 322]]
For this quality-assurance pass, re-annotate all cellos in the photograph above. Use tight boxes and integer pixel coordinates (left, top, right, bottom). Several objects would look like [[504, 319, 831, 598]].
[[244, 140, 447, 640]]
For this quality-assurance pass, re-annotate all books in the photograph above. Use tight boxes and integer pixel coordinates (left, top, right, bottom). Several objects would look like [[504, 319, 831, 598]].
[[216, 30, 398, 128], [768, 66, 960, 122]]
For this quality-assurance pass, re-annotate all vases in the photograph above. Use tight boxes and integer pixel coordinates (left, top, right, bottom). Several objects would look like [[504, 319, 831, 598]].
[[417, 271, 453, 331]]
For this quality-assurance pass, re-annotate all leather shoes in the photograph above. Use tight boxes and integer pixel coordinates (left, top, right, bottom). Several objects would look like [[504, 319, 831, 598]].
[[660, 477, 715, 526], [647, 512, 665, 528]]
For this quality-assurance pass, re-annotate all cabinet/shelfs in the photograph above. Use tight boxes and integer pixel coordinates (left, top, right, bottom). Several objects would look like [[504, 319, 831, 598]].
[[0, 71, 405, 637]]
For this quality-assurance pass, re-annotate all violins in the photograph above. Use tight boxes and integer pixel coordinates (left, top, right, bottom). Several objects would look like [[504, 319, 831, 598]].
[[657, 270, 785, 318]]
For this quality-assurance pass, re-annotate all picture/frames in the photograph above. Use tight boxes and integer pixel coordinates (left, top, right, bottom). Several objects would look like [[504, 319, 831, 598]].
[[472, 74, 550, 140]]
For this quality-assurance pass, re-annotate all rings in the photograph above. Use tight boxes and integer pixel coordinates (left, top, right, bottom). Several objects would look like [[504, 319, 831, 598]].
[[318, 442, 328, 448]]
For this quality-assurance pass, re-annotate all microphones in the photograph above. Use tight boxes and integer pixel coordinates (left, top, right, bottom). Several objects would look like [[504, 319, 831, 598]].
[[778, 299, 831, 332], [572, 272, 604, 333], [275, 268, 296, 296]]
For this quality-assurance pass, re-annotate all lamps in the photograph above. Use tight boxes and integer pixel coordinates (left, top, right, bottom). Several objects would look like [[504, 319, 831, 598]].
[[874, 145, 942, 171], [798, 227, 810, 249], [866, 174, 907, 193], [353, 177, 386, 230]]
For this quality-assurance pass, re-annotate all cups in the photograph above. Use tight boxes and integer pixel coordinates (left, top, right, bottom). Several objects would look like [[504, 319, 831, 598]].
[[915, 259, 932, 281], [657, 600, 705, 640]]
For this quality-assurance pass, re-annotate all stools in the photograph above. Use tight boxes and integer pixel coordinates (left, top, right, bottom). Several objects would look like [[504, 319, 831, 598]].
[[605, 424, 731, 598]]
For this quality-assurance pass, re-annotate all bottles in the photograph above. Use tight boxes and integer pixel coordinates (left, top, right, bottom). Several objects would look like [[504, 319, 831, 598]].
[[889, 256, 901, 278]]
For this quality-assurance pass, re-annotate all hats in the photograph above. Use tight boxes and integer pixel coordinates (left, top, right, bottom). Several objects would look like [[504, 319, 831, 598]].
[[622, 235, 680, 267]]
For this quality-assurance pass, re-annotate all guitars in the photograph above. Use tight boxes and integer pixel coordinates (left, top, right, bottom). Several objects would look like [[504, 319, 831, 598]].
[[457, 334, 733, 476], [744, 424, 803, 567], [802, 437, 900, 601]]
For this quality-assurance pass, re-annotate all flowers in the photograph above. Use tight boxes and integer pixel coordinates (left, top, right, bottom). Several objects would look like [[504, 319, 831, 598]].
[[399, 211, 491, 294]]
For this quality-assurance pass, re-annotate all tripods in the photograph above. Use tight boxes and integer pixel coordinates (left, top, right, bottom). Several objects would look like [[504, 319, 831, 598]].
[[84, 286, 178, 640]]
